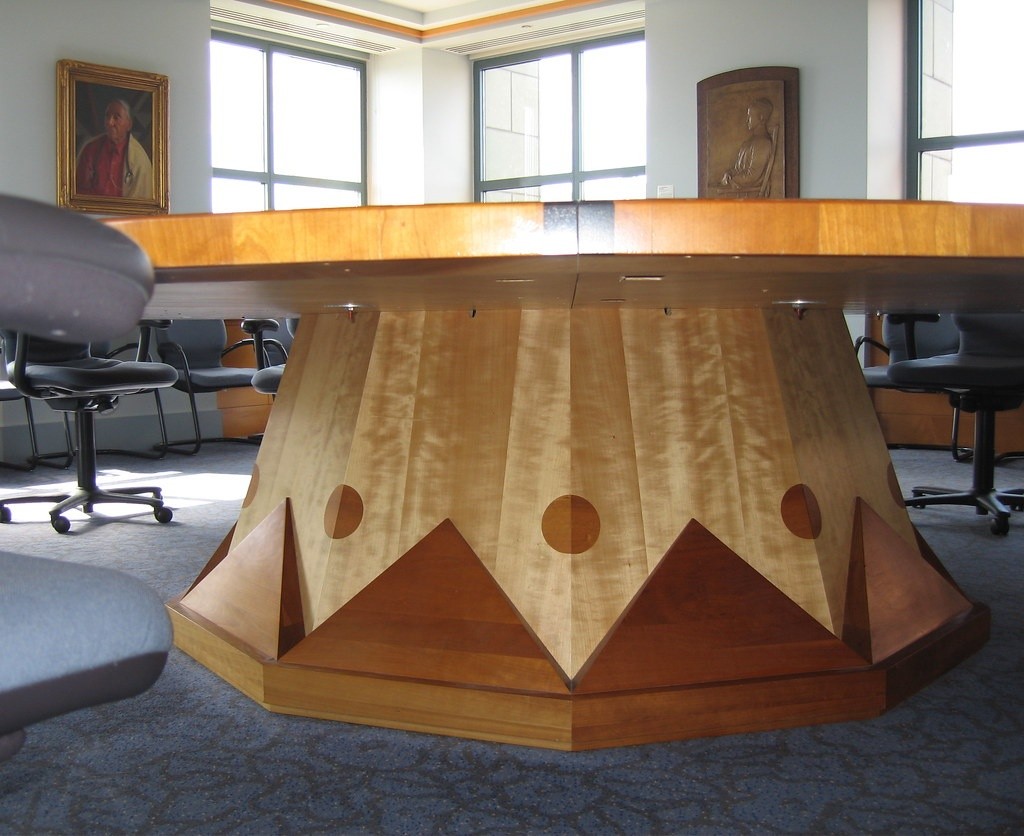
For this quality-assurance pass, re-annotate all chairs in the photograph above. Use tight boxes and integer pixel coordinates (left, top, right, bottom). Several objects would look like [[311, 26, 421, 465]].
[[0, 193, 297, 532], [856, 312, 1024, 537]]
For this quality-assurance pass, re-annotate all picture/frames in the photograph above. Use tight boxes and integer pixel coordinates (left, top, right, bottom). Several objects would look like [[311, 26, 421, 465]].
[[53, 58, 172, 216], [694, 66, 801, 200]]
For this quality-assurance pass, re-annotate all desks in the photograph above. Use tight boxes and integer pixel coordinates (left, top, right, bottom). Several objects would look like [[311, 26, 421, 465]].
[[104, 198, 1024, 752]]
